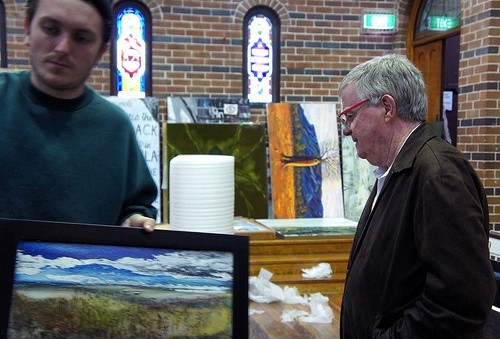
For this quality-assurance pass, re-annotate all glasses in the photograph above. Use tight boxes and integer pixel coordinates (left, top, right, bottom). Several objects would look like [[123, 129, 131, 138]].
[[339, 93, 397, 124]]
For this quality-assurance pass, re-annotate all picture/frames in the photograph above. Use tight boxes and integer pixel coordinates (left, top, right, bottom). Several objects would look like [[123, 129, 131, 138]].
[[0, 217, 250, 339]]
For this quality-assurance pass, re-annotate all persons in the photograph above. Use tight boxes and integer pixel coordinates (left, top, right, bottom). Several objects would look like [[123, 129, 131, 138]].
[[0, 0, 157, 233], [337, 53, 497, 339]]
